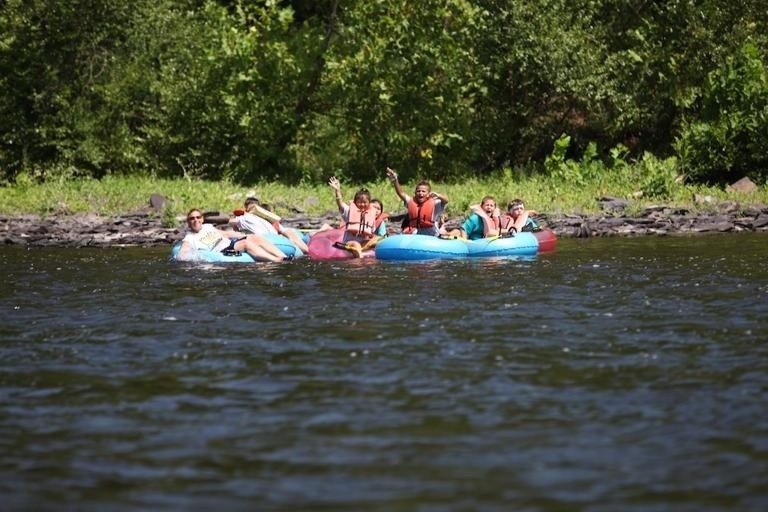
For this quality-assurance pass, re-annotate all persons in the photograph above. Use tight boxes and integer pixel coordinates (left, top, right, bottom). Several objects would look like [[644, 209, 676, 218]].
[[229, 197, 309, 256], [328, 176, 390, 260], [451, 195, 539, 241], [180, 209, 293, 263], [387, 167, 448, 237]]
